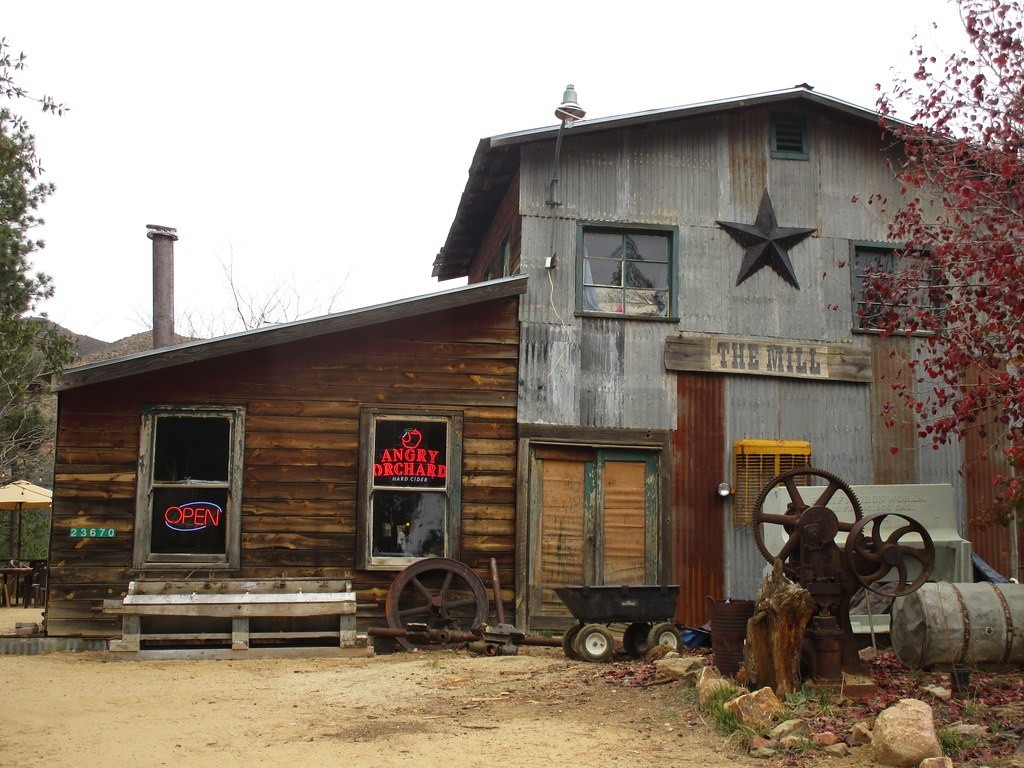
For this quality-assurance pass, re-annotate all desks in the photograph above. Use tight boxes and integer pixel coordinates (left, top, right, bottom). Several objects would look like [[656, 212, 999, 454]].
[[0, 568, 33, 607]]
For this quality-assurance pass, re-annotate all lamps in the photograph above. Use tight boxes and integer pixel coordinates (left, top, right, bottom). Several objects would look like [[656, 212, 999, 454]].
[[547, 84, 586, 206]]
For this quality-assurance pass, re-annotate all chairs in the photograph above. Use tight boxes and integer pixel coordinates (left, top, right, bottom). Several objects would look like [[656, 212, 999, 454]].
[[0, 560, 49, 609]]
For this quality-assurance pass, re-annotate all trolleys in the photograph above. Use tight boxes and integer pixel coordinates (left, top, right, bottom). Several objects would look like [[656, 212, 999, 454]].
[[550, 583, 712, 664]]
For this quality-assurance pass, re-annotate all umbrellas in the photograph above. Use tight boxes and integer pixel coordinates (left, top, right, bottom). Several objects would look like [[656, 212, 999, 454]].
[[0, 479, 53, 606]]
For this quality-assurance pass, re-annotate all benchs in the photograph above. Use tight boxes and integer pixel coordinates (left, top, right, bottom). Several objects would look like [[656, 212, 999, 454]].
[[102, 576, 355, 652]]
